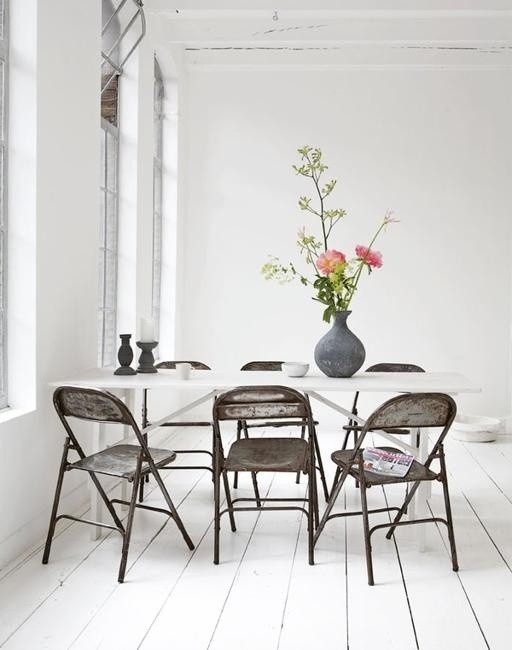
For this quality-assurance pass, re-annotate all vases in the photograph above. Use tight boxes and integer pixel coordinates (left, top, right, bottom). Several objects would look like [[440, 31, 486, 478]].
[[315, 311, 366, 377]]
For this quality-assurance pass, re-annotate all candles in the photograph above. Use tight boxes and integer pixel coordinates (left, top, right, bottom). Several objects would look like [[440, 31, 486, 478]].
[[141, 316, 154, 343]]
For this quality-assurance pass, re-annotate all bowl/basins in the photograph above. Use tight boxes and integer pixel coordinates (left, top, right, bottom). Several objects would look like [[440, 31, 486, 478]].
[[281, 362, 309, 377], [450, 415, 500, 442]]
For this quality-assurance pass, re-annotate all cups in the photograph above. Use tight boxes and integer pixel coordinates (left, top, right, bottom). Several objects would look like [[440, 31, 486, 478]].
[[175, 363, 195, 381]]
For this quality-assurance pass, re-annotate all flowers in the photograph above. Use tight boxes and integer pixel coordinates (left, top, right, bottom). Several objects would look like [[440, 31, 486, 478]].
[[261, 144, 402, 323]]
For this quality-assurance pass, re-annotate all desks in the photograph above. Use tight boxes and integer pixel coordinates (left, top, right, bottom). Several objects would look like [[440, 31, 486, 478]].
[[48, 368, 482, 553]]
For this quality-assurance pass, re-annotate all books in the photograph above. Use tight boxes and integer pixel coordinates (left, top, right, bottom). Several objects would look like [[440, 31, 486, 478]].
[[351, 445, 414, 477]]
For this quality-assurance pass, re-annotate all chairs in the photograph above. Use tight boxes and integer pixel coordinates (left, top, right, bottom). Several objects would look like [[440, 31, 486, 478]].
[[331, 363, 428, 513], [212, 385, 319, 565], [41, 385, 197, 582], [233, 361, 331, 507], [313, 392, 459, 586], [139, 360, 218, 502]]
